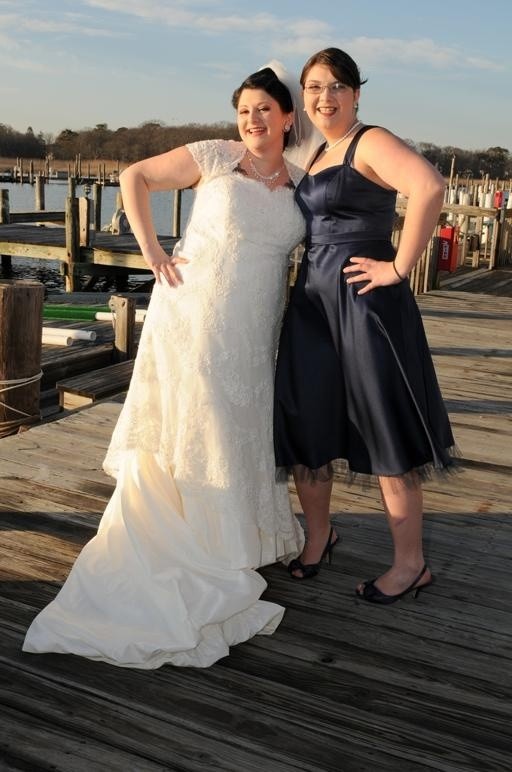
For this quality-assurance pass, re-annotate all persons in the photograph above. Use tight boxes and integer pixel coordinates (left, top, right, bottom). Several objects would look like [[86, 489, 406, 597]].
[[21, 59, 326, 671], [272, 45, 466, 605]]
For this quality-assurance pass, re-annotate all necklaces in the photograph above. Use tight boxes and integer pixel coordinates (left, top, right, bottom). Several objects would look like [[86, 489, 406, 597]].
[[246, 151, 284, 180], [324, 120, 361, 152]]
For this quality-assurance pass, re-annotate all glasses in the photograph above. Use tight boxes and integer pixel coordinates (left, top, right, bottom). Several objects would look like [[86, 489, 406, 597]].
[[304, 80, 351, 94]]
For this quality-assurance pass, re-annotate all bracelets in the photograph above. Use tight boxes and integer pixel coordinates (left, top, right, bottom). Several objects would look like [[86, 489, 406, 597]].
[[392, 259, 409, 282]]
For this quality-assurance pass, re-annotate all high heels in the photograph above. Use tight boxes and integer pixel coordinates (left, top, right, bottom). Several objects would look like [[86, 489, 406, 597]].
[[354, 564, 434, 606], [288, 525, 340, 581]]
